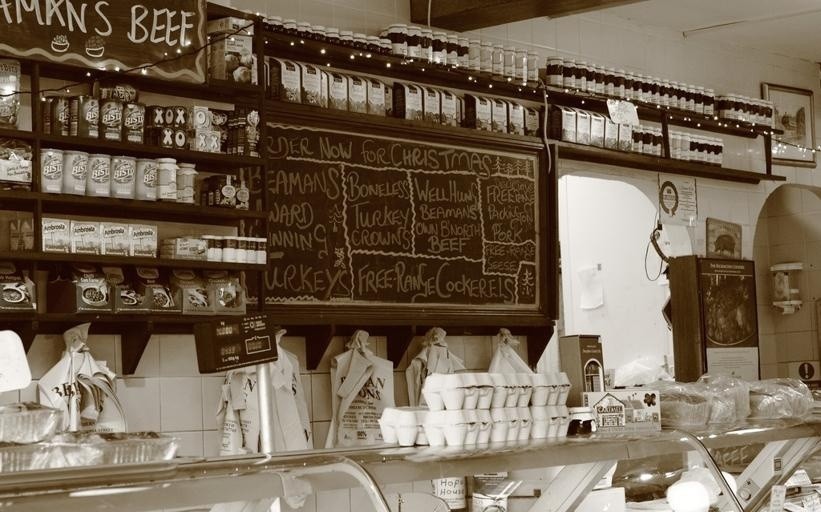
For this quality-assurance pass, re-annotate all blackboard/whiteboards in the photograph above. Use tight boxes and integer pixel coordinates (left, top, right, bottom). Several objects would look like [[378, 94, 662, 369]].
[[238, 104, 560, 326]]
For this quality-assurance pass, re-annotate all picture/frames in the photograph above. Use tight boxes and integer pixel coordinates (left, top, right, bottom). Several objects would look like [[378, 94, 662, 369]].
[[761, 82, 817, 168]]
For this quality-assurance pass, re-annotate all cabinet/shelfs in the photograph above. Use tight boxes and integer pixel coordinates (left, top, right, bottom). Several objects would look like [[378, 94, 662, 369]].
[[1, 54, 269, 334], [0, 399, 820, 512], [265, 29, 786, 184]]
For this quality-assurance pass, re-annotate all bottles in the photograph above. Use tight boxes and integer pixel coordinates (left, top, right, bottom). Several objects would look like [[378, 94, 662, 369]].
[[717, 91, 775, 131], [633, 126, 723, 168], [468, 39, 540, 82], [546, 56, 718, 119], [258, 12, 468, 70]]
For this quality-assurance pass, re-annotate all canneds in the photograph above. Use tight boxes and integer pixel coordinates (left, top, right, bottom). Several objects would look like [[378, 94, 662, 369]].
[[40, 79, 157, 202], [431, 477, 466, 510], [201, 175, 250, 212], [472, 493, 508, 512], [201, 235, 268, 264]]
